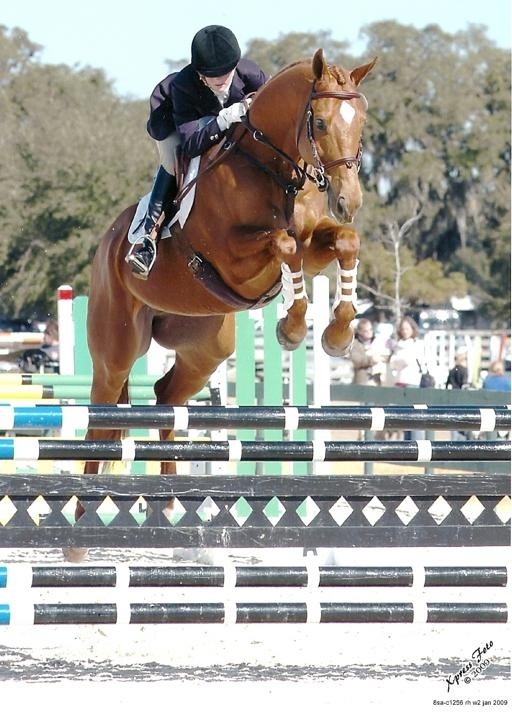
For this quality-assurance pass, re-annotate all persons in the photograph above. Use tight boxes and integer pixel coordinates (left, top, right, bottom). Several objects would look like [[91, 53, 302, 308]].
[[371, 322, 391, 378], [388, 317, 426, 390], [127, 26, 270, 282], [483, 361, 508, 391], [500, 335, 510, 374], [26, 322, 62, 373], [347, 321, 374, 385], [446, 351, 470, 389]]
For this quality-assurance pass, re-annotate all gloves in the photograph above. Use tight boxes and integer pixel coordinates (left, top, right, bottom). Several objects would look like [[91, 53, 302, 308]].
[[216, 102, 248, 131]]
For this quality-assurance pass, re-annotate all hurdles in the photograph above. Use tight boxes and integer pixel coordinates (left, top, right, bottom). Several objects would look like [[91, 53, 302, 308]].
[[1, 374, 510, 624]]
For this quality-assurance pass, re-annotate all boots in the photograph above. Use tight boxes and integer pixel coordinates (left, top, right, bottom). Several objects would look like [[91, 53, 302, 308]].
[[134, 165, 176, 276]]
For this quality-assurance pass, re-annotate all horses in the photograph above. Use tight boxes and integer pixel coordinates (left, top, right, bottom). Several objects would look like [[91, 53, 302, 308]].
[[61, 47, 379, 565]]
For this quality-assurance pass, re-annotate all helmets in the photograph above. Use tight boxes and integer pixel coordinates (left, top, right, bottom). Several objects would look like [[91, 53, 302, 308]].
[[191, 26, 240, 78]]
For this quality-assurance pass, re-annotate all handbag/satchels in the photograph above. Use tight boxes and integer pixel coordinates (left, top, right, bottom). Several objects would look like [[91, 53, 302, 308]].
[[421, 374, 435, 388]]
